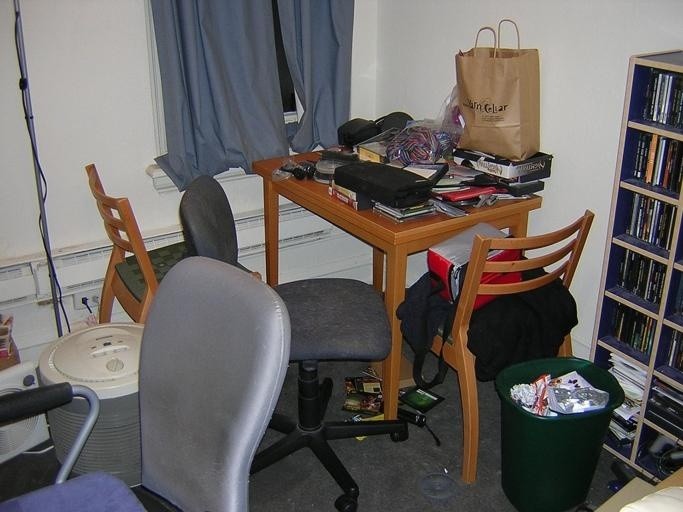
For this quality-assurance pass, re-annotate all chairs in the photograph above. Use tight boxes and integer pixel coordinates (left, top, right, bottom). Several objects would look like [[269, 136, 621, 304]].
[[0, 257, 291, 512], [406, 209, 594, 484], [85, 164, 262, 324], [179, 174, 409, 512]]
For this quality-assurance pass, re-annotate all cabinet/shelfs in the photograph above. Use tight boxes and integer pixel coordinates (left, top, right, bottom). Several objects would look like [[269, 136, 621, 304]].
[[590, 50, 683, 484]]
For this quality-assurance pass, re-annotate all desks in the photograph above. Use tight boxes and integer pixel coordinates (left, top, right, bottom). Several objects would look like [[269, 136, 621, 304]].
[[253, 146, 542, 420]]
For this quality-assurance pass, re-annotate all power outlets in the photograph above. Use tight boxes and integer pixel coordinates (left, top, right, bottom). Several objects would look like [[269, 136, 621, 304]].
[[73, 290, 100, 309]]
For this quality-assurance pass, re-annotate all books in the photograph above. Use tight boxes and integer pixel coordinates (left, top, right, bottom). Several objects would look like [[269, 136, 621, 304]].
[[328, 180, 437, 219], [608, 71, 683, 443]]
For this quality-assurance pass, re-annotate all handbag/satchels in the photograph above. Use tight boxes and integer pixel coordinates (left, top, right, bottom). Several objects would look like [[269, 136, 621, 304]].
[[455, 19, 540, 161], [338, 112, 413, 144], [335, 162, 432, 208], [427, 221, 523, 312]]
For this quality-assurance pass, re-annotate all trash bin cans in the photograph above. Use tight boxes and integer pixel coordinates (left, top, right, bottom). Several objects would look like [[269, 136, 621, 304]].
[[494, 356, 625, 512]]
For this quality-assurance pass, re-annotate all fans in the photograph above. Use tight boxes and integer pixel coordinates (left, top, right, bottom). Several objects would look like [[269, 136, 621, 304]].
[[0, 361, 50, 464]]
